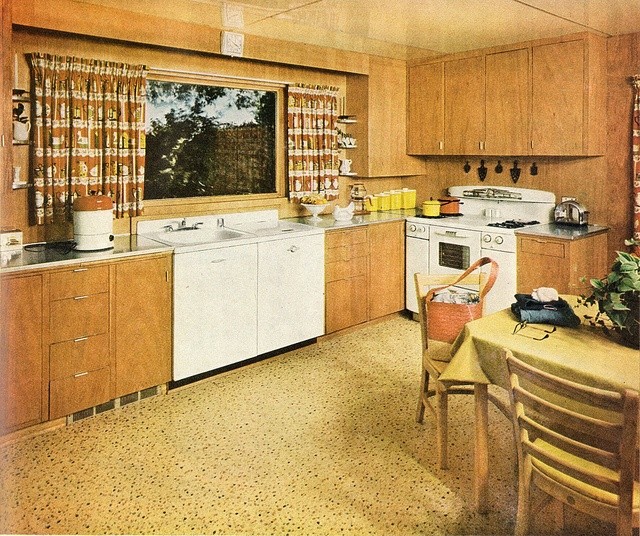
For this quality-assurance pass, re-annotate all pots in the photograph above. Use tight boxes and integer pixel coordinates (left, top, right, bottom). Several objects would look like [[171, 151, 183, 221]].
[[419, 196, 441, 217], [437, 192, 466, 214]]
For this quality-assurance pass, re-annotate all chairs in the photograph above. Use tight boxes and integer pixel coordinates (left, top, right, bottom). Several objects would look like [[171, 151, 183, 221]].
[[501, 348, 639, 536], [414, 273, 521, 470]]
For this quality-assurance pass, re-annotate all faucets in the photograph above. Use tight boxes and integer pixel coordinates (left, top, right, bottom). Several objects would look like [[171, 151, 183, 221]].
[[177, 218, 188, 231]]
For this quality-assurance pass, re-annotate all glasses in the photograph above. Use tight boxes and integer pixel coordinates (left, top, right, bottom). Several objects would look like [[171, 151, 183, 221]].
[[513, 319, 556, 341]]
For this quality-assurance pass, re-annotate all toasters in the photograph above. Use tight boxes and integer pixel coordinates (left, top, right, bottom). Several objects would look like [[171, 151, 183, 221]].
[[553, 200, 590, 227]]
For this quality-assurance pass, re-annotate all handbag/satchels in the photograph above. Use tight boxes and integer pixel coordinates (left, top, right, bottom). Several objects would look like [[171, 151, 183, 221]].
[[424, 257, 499, 362]]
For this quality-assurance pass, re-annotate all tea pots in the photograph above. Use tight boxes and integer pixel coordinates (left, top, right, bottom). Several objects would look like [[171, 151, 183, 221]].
[[338, 157, 352, 175], [347, 183, 367, 199], [12, 120, 31, 142]]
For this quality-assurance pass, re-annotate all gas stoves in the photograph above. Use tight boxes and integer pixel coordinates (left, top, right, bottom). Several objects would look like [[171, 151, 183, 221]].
[[404, 213, 556, 236]]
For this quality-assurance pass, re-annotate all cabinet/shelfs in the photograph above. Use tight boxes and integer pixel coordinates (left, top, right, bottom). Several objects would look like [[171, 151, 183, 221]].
[[13, 90, 33, 189], [336, 120, 359, 176], [326, 221, 405, 334], [516, 231, 608, 295], [1, 269, 43, 436], [172, 232, 326, 382], [113, 253, 173, 398], [47, 262, 111, 422], [485, 31, 600, 156], [406, 48, 485, 155]]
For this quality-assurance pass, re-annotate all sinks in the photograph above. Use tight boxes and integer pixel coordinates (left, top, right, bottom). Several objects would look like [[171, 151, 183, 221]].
[[159, 228, 240, 245]]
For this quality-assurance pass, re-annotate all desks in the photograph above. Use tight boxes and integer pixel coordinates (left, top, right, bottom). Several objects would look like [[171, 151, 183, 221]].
[[465, 295, 640, 515]]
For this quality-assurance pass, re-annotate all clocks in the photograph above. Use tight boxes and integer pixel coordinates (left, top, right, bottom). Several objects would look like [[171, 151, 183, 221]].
[[219, 3, 244, 29], [220, 31, 244, 56]]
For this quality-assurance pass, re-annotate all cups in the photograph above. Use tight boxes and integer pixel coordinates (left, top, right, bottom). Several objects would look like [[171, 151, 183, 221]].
[[12, 167, 21, 184]]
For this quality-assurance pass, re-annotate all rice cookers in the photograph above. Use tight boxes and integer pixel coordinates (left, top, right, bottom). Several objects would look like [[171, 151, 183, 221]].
[[72, 190, 115, 254]]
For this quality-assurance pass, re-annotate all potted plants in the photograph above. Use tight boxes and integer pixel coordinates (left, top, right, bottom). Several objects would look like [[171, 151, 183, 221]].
[[574, 238, 640, 345], [13, 103, 31, 140]]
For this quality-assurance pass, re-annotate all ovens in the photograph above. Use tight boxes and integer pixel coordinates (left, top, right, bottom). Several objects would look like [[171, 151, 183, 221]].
[[398, 236, 517, 322]]
